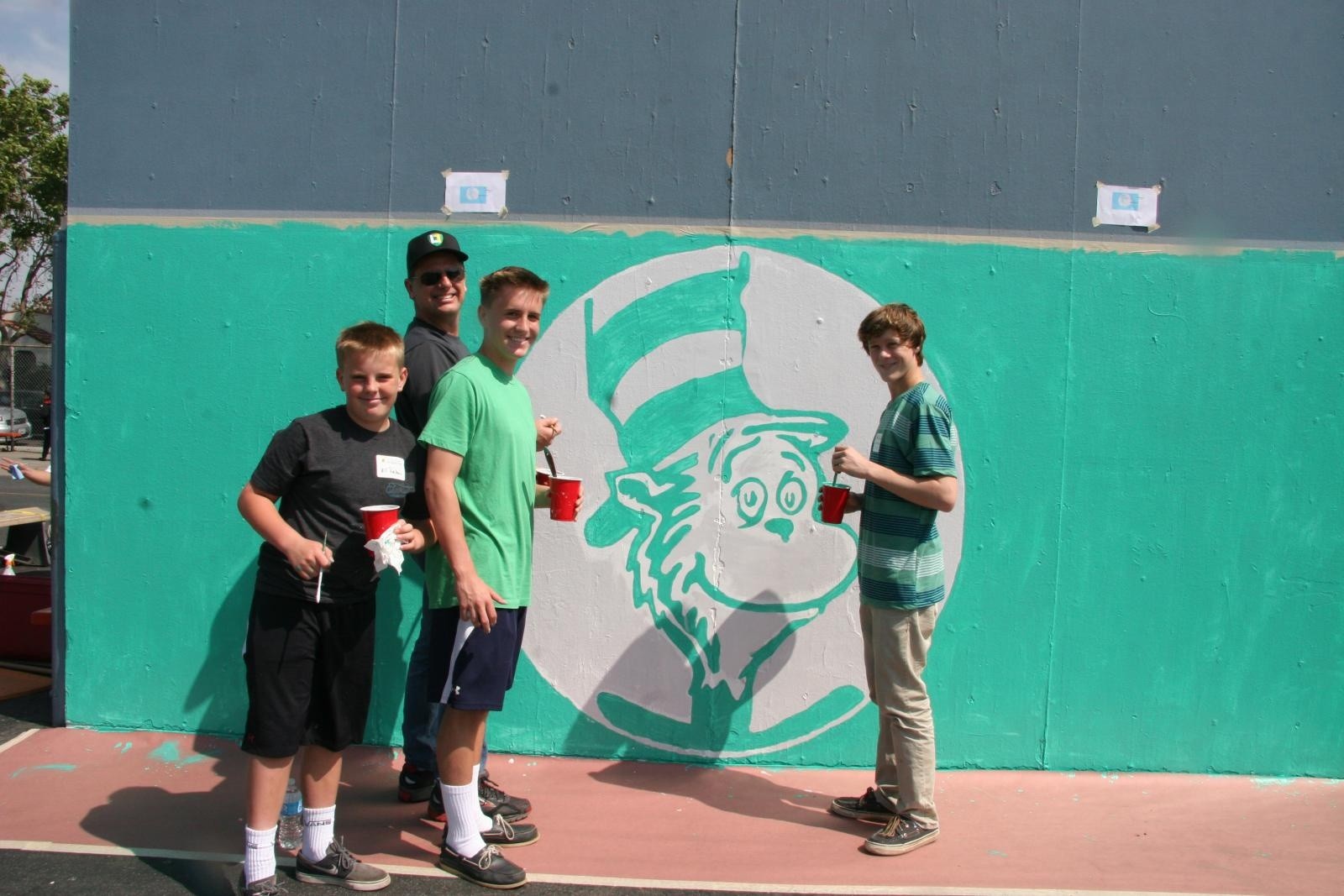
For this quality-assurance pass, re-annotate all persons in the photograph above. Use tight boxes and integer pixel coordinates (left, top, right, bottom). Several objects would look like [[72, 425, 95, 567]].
[[418, 267, 583, 888], [236, 320, 439, 896], [395, 232, 565, 822], [38, 391, 52, 461], [832, 303, 958, 857], [0, 456, 51, 486]]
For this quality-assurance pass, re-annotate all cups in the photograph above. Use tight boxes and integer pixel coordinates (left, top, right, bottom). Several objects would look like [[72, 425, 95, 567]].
[[820, 481, 852, 525], [549, 475, 582, 522], [360, 504, 401, 558]]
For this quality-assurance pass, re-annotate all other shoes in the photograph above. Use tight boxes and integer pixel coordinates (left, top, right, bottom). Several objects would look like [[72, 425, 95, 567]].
[[39, 457, 47, 461]]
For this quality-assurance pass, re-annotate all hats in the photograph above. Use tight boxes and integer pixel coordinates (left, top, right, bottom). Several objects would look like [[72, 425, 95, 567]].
[[406, 230, 469, 271]]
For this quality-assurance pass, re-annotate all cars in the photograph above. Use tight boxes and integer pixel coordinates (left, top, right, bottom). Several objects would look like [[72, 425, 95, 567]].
[[0, 389, 50, 437], [0, 402, 33, 443]]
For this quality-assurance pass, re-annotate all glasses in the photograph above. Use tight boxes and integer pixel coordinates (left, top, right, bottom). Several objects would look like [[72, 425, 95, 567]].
[[410, 267, 467, 286]]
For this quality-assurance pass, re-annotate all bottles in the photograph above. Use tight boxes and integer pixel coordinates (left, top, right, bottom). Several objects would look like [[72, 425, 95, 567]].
[[2, 554, 16, 576]]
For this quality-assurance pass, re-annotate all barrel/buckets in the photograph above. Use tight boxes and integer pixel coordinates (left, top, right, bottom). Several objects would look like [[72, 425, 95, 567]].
[[0, 574, 51, 665]]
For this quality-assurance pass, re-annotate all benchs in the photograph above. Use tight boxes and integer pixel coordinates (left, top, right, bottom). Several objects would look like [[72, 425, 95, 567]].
[[0, 506, 51, 567]]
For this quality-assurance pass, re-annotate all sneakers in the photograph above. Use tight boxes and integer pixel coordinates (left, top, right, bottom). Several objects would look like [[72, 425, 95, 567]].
[[397, 762, 490, 803], [864, 815, 941, 856], [427, 775, 533, 823], [236, 866, 291, 896], [294, 835, 391, 891], [441, 812, 541, 847], [830, 787, 896, 823], [438, 838, 528, 889]]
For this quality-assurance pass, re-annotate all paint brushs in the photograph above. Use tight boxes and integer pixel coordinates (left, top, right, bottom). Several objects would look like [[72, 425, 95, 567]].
[[540, 415, 557, 433], [315, 531, 328, 603]]
